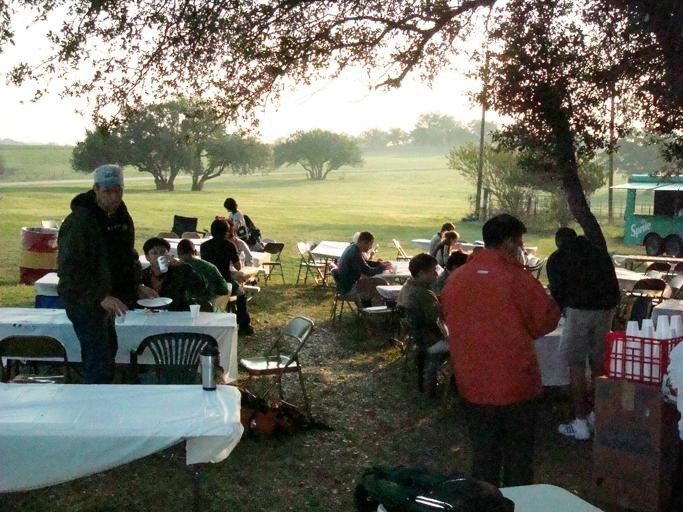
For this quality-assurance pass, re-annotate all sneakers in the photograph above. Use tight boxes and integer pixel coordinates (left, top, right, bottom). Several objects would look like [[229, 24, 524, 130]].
[[558, 415, 595, 440], [239, 325, 253, 334]]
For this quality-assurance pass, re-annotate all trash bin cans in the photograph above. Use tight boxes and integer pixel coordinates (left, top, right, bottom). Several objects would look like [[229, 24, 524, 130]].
[[18, 226, 59, 285]]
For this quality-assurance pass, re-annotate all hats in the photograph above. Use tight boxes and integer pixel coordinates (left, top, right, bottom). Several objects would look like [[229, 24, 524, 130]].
[[93, 165, 124, 188]]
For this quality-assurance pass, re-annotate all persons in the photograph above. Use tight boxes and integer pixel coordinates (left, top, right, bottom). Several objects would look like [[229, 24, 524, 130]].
[[546, 225, 620, 441], [440, 212, 561, 488], [137, 195, 263, 335], [336, 221, 480, 356], [58, 164, 159, 385]]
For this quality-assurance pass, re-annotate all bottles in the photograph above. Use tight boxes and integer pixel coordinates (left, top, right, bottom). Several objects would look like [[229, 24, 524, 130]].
[[239, 252, 246, 269]]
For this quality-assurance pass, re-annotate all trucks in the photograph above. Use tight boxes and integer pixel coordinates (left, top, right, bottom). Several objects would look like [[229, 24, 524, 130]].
[[609, 174, 682, 257]]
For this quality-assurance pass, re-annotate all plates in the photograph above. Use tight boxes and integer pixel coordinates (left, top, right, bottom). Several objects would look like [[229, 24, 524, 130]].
[[136, 298, 173, 307]]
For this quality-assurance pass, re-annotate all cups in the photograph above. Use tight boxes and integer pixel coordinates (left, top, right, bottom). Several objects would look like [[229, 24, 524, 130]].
[[608, 314, 682, 383], [189, 305, 201, 318], [157, 256, 168, 273], [114, 309, 126, 324], [200, 346, 218, 390]]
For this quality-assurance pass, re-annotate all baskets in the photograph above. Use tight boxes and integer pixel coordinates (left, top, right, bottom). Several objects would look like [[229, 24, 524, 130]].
[[604, 329, 683, 385]]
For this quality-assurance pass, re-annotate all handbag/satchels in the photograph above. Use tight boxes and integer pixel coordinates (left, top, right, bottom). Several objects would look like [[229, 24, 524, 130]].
[[355, 465, 515, 512], [238, 387, 304, 436]]
